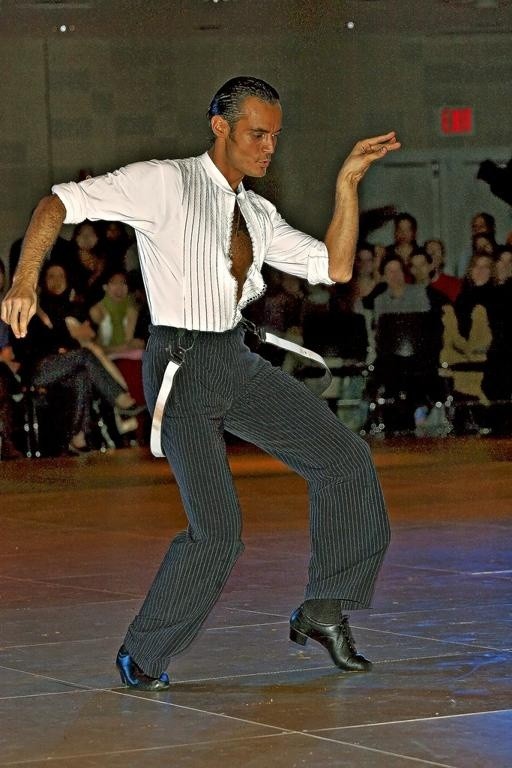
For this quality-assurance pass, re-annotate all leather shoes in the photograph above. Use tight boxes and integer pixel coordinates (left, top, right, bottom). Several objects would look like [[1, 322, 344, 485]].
[[116, 645, 171, 692], [288, 606, 372, 672]]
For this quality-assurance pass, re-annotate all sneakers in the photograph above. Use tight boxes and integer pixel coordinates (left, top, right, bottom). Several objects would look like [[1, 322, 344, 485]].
[[66, 397, 146, 456]]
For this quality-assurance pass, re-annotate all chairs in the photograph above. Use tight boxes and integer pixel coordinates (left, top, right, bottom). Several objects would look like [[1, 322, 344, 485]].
[[305, 313, 445, 439]]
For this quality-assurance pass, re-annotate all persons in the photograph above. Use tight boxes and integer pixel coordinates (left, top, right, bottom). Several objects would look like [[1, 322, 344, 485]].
[[0, 77, 403, 691]]
